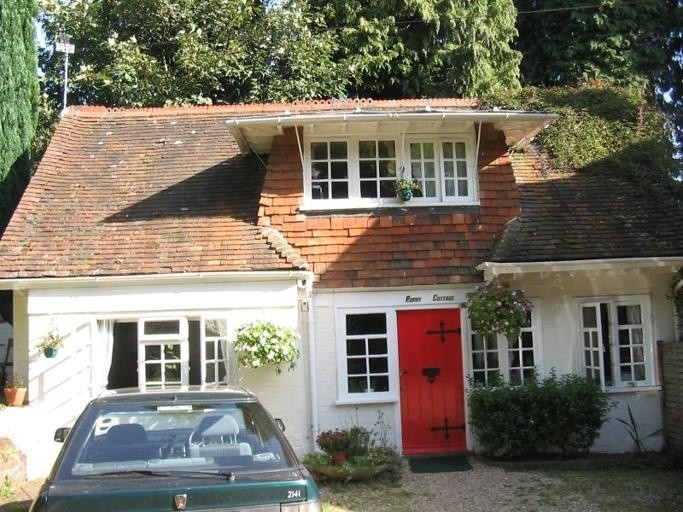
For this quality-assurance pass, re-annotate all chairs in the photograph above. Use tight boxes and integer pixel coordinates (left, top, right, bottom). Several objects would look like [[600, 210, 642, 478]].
[[96, 424, 160, 461], [188, 415, 252, 459]]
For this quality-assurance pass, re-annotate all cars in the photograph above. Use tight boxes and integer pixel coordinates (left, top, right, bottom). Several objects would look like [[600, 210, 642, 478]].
[[27, 387, 324, 512]]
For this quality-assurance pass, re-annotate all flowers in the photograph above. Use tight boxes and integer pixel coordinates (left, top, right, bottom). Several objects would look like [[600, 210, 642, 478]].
[[232, 321, 301, 374], [458, 276, 534, 347]]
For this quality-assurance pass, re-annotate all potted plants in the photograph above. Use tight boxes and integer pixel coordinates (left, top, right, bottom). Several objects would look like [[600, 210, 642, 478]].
[[315, 428, 350, 452], [4, 373, 26, 407], [34, 330, 64, 358], [392, 179, 423, 201], [348, 424, 370, 467]]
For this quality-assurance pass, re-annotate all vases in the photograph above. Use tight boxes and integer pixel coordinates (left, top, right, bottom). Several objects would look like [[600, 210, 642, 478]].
[[332, 451, 347, 464]]
